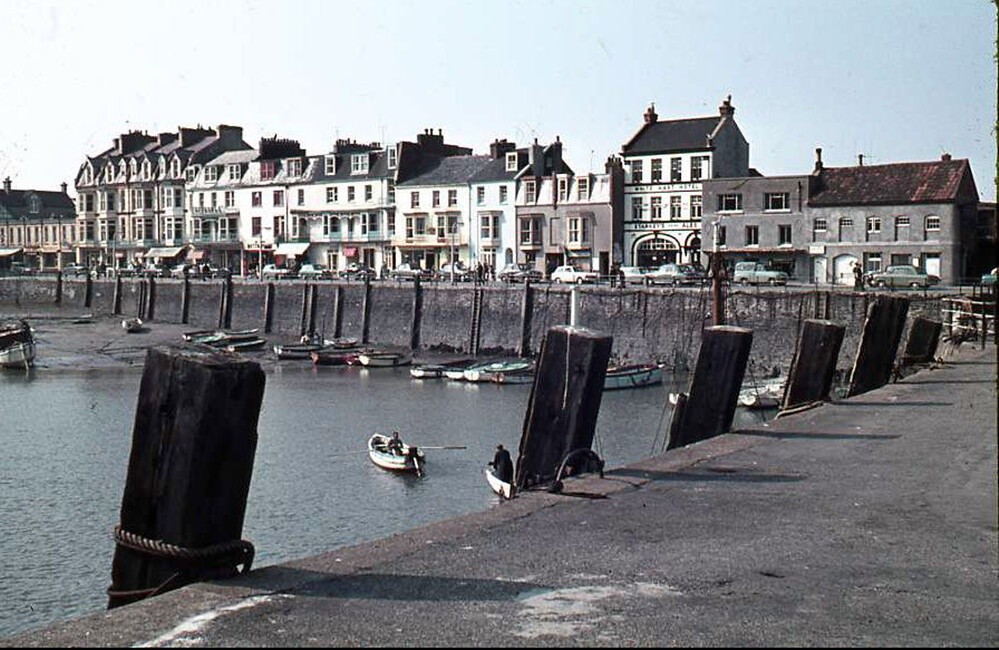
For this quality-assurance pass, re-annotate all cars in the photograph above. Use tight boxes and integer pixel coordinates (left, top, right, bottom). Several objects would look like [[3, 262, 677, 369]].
[[262, 260, 471, 282], [499, 263, 542, 284], [733, 261, 787, 287], [643, 263, 704, 287], [865, 263, 940, 290], [551, 266, 601, 282]]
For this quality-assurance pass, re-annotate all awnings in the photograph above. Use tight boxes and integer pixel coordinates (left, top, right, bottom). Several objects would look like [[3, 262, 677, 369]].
[[273, 244, 311, 254], [0, 248, 24, 258], [144, 247, 185, 258]]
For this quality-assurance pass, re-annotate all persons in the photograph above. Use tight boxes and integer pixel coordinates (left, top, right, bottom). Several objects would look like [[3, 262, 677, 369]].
[[387, 431, 404, 456], [492, 444, 513, 483], [74, 262, 233, 280], [358, 262, 494, 285], [853, 263, 867, 291], [508, 260, 626, 287]]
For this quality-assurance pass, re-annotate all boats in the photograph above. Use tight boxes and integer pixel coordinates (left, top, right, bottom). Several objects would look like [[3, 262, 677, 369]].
[[0, 320, 36, 368], [272, 342, 330, 357], [602, 362, 663, 389], [367, 432, 425, 477], [358, 353, 415, 368], [408, 357, 537, 383], [182, 329, 268, 352], [309, 347, 376, 365], [123, 319, 145, 333]]
